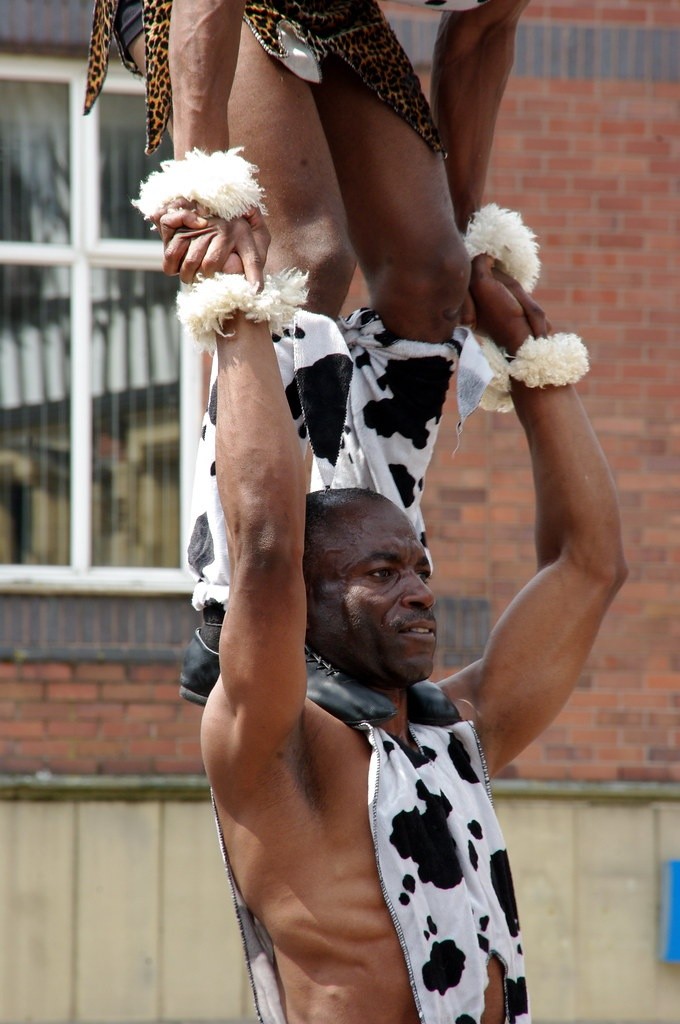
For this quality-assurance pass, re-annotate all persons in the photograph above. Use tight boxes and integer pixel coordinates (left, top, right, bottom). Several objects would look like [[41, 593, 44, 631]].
[[148, 196, 628, 1024]]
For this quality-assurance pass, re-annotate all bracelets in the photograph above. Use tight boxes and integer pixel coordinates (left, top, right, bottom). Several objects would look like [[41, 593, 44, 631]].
[[474, 331, 598, 410], [169, 268, 315, 348], [462, 201, 543, 294], [83, 0, 530, 728], [129, 147, 272, 226]]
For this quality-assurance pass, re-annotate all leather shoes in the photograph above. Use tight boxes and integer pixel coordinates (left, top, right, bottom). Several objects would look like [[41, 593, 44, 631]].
[[177, 627, 397, 728], [407, 679, 461, 726]]
